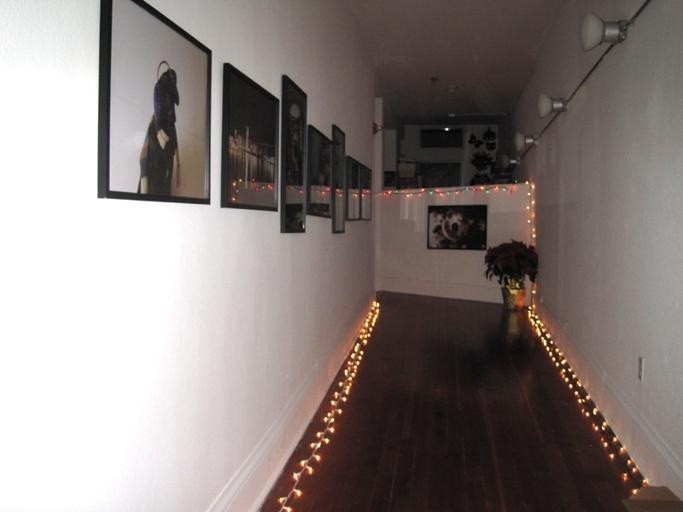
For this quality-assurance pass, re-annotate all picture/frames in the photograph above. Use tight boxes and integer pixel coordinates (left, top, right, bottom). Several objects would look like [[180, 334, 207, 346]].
[[427, 204, 488, 251], [221, 62, 373, 234], [99, 0, 213, 204]]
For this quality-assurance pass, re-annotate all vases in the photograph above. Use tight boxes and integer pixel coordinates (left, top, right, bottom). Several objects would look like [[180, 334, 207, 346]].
[[501, 278, 526, 311]]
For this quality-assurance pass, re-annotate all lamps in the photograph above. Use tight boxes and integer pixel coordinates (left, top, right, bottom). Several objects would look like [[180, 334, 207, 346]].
[[498, 10, 629, 172]]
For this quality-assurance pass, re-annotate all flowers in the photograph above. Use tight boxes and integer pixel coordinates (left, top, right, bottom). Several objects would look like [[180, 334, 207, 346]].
[[482, 238, 539, 283]]
